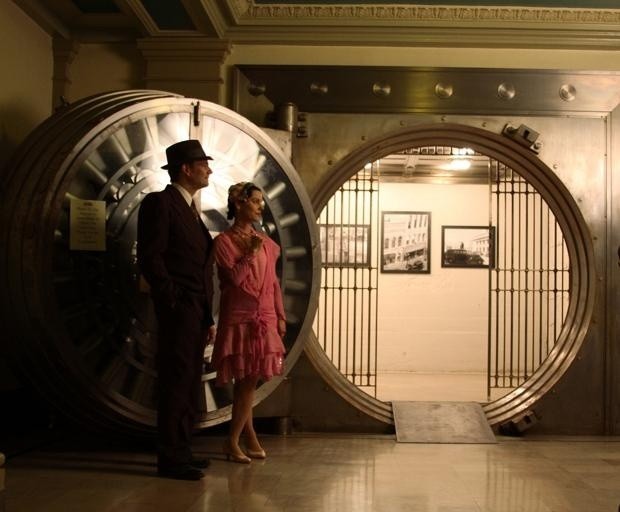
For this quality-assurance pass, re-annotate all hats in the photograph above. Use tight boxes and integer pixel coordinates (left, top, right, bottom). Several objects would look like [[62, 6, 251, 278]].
[[161, 140, 213, 169]]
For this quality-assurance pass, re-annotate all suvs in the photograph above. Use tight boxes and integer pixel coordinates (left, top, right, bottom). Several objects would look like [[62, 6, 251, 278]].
[[409, 260, 425, 270], [444, 248, 470, 264]]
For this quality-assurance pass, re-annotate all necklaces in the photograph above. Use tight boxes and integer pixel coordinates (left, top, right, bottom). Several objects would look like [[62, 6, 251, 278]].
[[232, 223, 253, 239]]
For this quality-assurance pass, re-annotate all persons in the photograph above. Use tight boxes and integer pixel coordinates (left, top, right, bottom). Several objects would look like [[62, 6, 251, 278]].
[[212, 182, 289, 464], [137, 138, 215, 480]]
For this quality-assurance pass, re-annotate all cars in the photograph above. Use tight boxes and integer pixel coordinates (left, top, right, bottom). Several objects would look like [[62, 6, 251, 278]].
[[464, 254, 484, 265]]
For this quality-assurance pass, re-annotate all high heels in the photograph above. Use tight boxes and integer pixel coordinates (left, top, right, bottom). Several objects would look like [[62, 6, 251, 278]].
[[223, 440, 266, 464]]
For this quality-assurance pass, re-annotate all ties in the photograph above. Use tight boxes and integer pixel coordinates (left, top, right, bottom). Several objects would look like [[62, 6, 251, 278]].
[[190, 199, 198, 221]]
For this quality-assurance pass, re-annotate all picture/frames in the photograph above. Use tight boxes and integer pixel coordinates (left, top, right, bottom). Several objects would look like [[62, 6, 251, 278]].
[[314, 200, 497, 274]]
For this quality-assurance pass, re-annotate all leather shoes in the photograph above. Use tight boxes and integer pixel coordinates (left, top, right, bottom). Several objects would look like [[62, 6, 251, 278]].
[[157, 457, 209, 479]]
